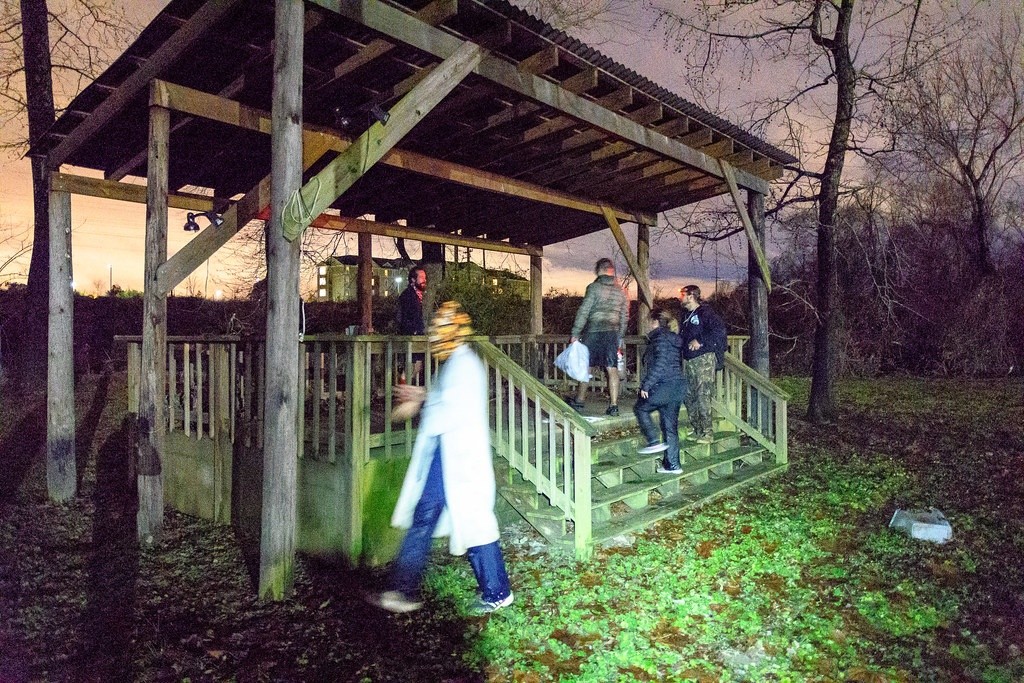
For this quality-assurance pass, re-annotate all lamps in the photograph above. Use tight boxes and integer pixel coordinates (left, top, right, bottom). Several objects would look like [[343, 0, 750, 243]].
[[333, 91, 395, 129], [183, 203, 225, 231]]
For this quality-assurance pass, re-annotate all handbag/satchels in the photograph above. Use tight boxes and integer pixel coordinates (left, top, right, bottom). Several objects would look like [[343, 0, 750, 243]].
[[553, 340, 590, 383]]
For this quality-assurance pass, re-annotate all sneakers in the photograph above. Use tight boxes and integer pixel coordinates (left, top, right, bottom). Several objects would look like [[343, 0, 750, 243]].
[[469, 592, 514, 613], [374, 590, 422, 613]]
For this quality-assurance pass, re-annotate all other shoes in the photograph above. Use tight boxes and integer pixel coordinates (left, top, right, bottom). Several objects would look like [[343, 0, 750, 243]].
[[657, 466, 683, 474], [697, 434, 713, 443], [637, 443, 666, 454], [606, 405, 619, 416], [567, 397, 583, 411], [686, 432, 701, 442]]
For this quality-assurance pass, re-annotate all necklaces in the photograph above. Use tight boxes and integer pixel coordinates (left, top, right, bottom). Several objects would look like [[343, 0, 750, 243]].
[[683, 305, 700, 324]]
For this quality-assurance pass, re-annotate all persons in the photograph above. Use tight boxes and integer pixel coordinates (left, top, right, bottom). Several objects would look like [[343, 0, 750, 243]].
[[398, 266, 426, 385], [370, 297, 514, 615], [633, 308, 688, 475], [679, 285, 726, 443], [566, 258, 630, 417]]
